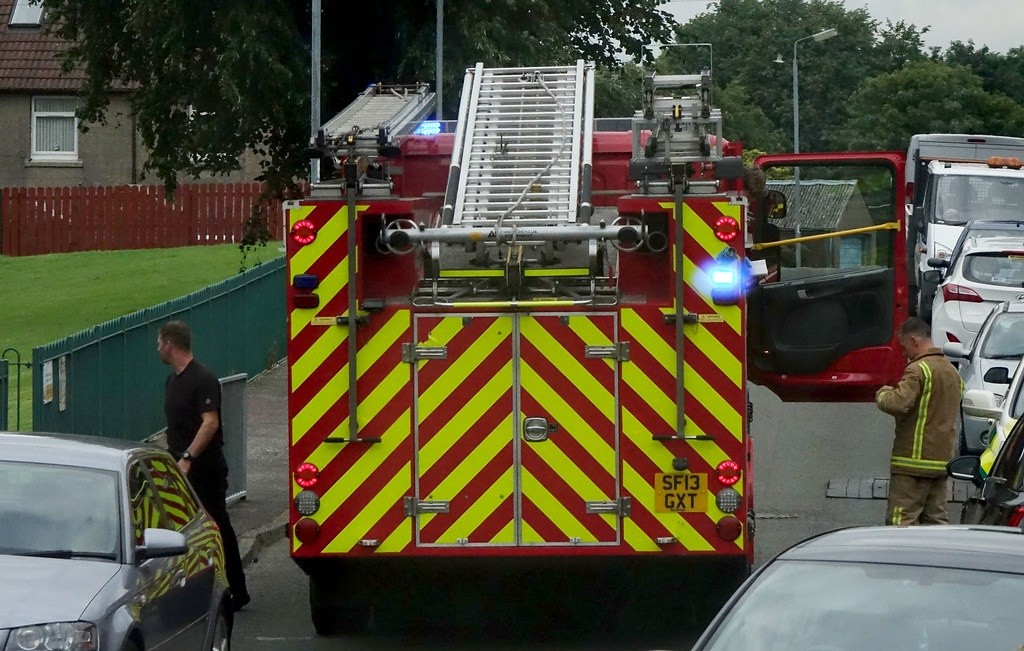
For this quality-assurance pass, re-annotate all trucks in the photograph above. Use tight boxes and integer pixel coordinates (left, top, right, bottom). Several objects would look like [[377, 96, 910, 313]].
[[899, 132, 1024, 322]]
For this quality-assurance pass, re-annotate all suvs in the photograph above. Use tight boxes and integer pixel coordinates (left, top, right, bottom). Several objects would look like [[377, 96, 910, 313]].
[[926, 218, 1024, 365], [942, 295, 1024, 457]]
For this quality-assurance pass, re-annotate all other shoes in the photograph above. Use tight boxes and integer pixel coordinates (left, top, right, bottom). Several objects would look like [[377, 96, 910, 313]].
[[231, 590, 251, 612]]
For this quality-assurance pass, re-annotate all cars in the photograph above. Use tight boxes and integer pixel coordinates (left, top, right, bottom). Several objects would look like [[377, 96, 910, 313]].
[[690, 524, 1024, 649], [2, 431, 237, 651], [946, 413, 1024, 531], [973, 357, 1024, 489]]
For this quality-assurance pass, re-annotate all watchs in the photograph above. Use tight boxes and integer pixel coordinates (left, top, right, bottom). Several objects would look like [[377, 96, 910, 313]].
[[182, 450, 194, 461]]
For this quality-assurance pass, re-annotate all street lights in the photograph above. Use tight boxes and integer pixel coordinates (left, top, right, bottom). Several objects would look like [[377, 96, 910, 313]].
[[790, 31, 845, 274]]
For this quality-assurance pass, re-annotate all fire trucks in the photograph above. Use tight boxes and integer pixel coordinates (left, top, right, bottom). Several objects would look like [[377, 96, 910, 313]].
[[276, 42, 916, 641]]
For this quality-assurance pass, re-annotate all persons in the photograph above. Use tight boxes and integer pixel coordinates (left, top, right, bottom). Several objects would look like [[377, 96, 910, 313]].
[[875, 317, 964, 528], [156, 321, 250, 612]]
